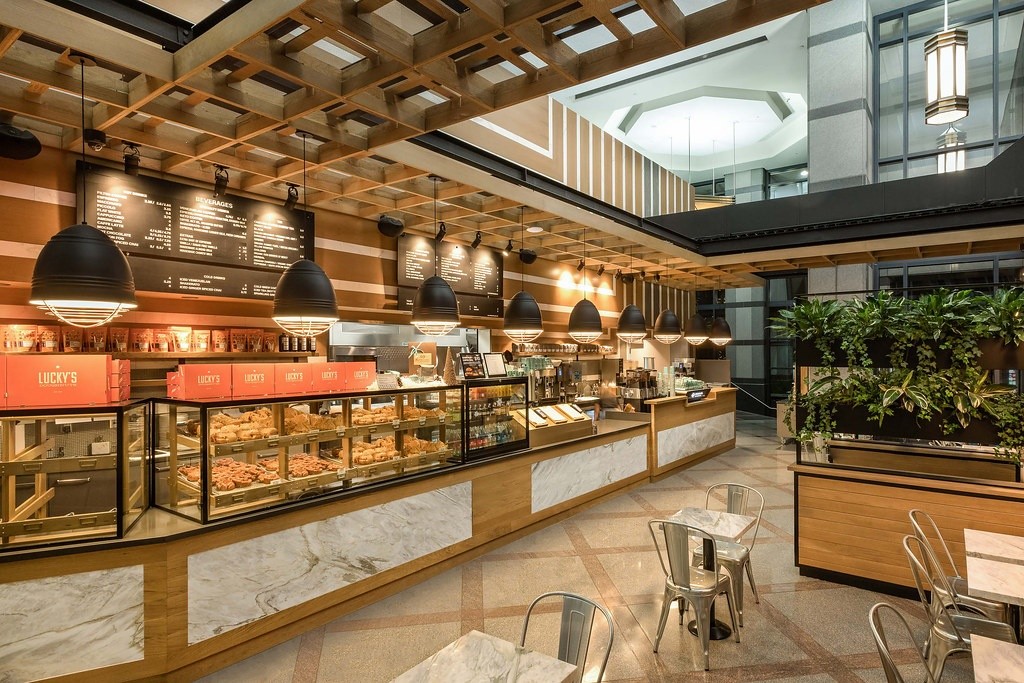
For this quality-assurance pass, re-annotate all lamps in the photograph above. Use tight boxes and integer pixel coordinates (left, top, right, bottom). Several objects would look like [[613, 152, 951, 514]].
[[937, 122, 966, 173], [922, 0, 970, 126], [0, 54, 733, 348]]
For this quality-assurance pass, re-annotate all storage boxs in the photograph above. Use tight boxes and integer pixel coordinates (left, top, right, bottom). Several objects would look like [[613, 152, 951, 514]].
[[0, 354, 378, 407]]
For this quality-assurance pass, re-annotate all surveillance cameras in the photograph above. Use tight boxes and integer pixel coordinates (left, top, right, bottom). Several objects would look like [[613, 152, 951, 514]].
[[84, 128, 106, 151]]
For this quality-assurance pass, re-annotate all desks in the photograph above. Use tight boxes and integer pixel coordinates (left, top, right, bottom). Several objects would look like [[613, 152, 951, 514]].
[[963, 526, 1024, 644], [970, 633, 1024, 683], [574, 396, 600, 420]]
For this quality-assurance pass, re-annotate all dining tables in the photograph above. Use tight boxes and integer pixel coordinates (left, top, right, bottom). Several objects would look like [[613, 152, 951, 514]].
[[658, 508, 757, 640], [389, 629, 579, 683]]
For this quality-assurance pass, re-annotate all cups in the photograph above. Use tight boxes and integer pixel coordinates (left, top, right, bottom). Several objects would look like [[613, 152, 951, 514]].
[[658, 366, 675, 398]]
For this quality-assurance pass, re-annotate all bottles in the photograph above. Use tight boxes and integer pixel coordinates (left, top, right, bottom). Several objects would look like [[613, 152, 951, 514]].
[[583, 382, 599, 397], [447, 402, 513, 456], [279, 332, 316, 351], [519, 344, 578, 353]]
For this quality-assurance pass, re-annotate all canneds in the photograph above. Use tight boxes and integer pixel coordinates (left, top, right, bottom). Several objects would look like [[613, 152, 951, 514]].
[[521, 343, 599, 352], [526, 357, 551, 369]]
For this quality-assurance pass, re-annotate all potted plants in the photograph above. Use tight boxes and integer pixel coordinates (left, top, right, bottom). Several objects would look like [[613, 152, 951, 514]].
[[762, 283, 1024, 471]]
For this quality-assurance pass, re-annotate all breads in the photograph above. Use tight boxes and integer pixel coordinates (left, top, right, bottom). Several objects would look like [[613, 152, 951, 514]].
[[179, 405, 448, 492]]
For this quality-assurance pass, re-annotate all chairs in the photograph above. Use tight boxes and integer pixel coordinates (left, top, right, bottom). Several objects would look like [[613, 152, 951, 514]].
[[519, 590, 615, 683], [647, 519, 740, 673], [685, 482, 764, 628], [868, 509, 1019, 683]]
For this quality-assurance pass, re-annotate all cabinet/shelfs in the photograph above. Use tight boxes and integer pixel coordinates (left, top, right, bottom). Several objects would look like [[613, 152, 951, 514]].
[[1, 281, 322, 387], [152, 376, 530, 551], [511, 342, 620, 361], [1, 395, 153, 545]]
